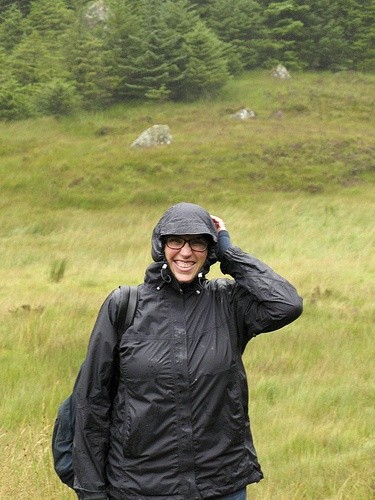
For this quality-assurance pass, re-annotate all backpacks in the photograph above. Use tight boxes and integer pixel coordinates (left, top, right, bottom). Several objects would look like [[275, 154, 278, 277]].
[[52, 286, 137, 488]]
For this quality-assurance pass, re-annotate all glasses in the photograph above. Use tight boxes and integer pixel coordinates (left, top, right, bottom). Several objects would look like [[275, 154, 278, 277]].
[[164, 236, 209, 252]]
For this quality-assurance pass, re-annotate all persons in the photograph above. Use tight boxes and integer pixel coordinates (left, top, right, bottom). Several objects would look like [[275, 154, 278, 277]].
[[51, 202, 302, 500]]
